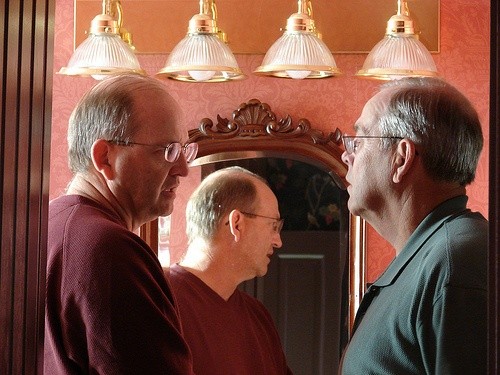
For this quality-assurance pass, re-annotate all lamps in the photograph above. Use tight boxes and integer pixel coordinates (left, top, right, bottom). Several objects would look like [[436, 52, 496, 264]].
[[58, 0, 147, 80], [158, 0, 249, 83], [355, 0, 439, 80], [253, 0, 342, 80]]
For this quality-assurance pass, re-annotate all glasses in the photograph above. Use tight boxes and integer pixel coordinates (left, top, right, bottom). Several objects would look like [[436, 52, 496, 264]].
[[107, 140, 198, 163], [225, 212, 285, 234], [341, 133, 419, 155]]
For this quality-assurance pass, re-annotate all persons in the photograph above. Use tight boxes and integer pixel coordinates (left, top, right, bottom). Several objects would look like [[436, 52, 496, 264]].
[[161, 166, 292, 375], [43, 74, 193, 375], [341, 75, 500, 375]]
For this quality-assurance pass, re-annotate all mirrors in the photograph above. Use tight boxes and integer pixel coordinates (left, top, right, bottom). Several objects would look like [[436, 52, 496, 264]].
[[141, 100, 367, 375]]
